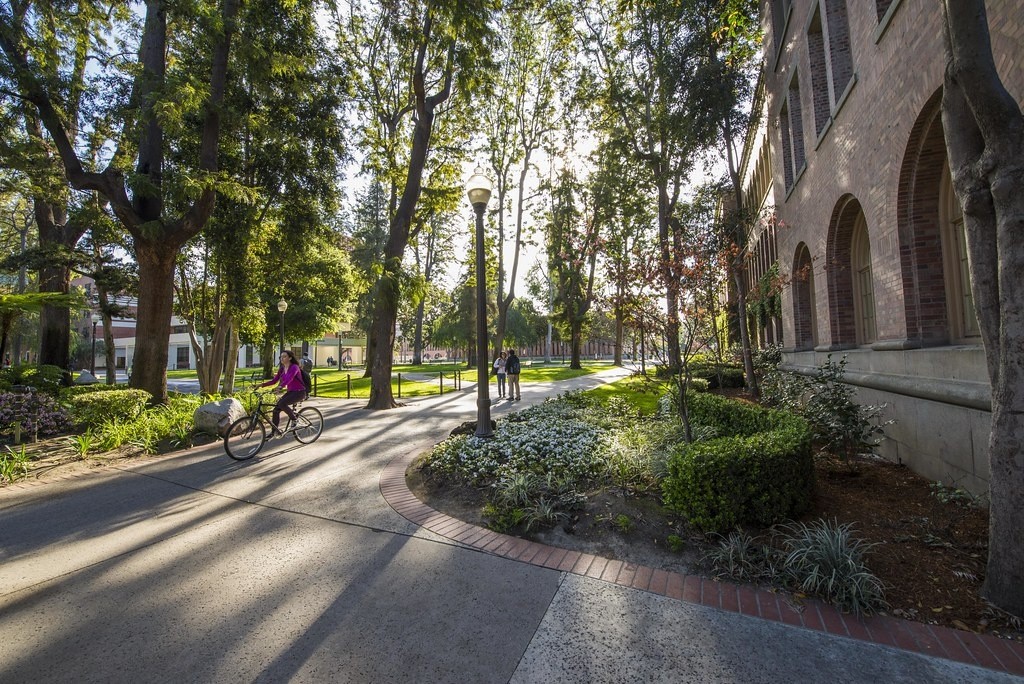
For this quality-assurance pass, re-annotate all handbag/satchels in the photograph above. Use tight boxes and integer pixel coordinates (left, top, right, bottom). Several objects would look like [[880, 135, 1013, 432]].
[[492, 367, 498, 375]]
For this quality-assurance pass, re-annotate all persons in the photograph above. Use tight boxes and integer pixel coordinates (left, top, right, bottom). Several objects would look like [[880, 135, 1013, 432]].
[[504, 349, 521, 401], [493, 351, 508, 399], [5, 357, 10, 373], [255, 349, 307, 442], [327, 356, 334, 367], [298, 352, 313, 401]]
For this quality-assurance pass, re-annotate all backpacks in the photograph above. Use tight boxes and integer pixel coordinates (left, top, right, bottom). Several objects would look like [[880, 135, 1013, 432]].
[[302, 358, 312, 372], [510, 362, 520, 373], [280, 364, 311, 393]]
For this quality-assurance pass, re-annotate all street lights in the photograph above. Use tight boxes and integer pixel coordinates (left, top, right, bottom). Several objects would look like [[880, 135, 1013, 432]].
[[91, 313, 99, 377], [277, 296, 288, 394], [464, 160, 496, 436]]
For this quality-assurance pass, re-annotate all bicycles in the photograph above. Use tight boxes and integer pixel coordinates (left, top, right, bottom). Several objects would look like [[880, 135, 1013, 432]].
[[224, 387, 324, 461]]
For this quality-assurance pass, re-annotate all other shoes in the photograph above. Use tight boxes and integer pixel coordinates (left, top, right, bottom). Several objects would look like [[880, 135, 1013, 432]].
[[291, 420, 299, 430], [506, 397, 514, 401], [265, 433, 274, 440]]
